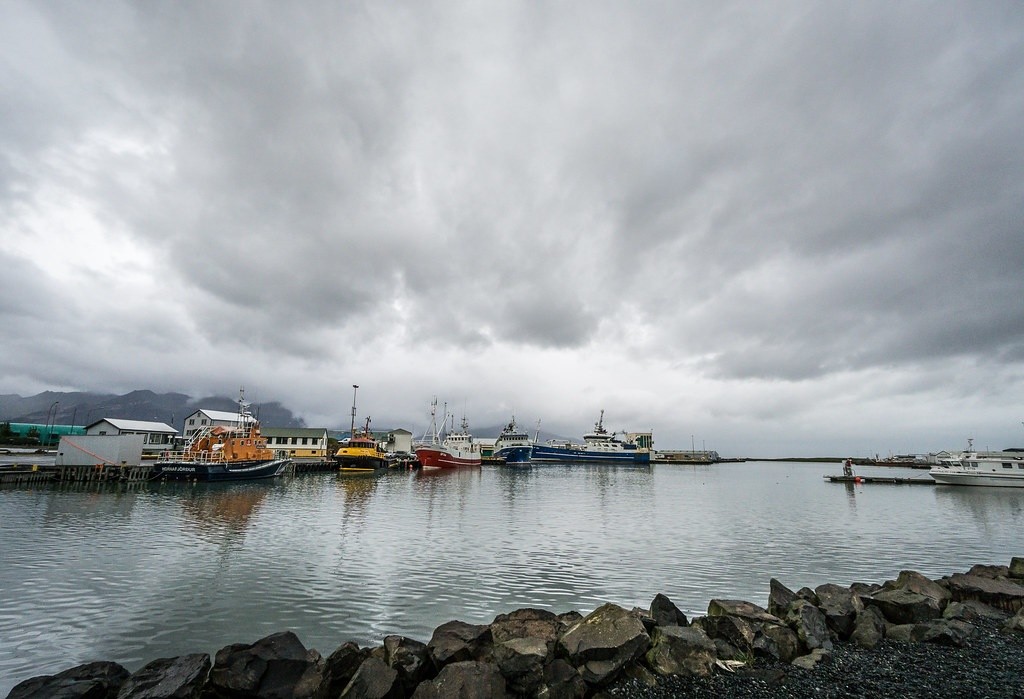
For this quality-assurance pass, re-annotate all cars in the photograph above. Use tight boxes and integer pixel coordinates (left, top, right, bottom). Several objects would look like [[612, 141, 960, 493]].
[[385, 449, 418, 461]]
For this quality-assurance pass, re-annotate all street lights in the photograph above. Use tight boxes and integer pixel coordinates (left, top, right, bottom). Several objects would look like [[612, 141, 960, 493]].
[[41, 401, 59, 450], [691, 435, 694, 457]]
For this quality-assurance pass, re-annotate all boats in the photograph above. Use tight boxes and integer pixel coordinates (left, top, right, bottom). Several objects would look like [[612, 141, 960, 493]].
[[529, 411, 650, 465], [151, 383, 294, 485], [864, 437, 1024, 488], [334, 384, 388, 472], [414, 393, 484, 471], [492, 414, 534, 466]]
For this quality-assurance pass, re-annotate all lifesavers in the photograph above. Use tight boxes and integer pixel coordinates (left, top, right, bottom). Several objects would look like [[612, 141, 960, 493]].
[[444, 442, 448, 446]]
